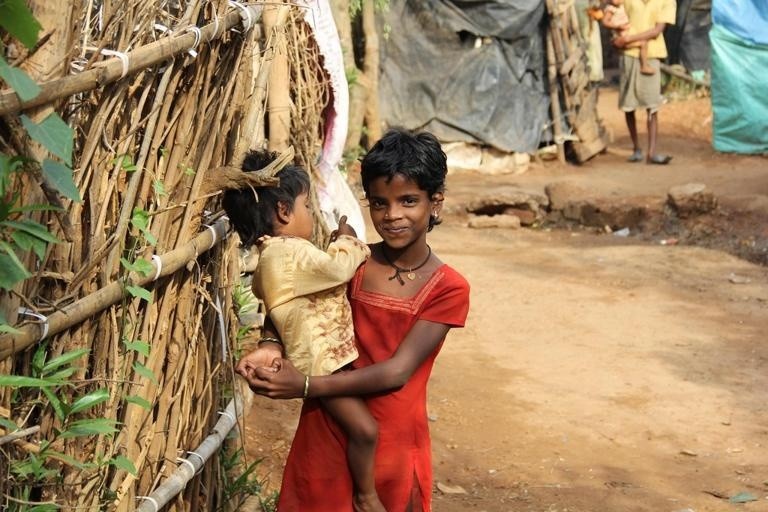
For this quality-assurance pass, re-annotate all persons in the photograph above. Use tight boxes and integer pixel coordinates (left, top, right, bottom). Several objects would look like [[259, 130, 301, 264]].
[[598, 0, 658, 76], [218, 146, 391, 511], [232, 129, 473, 511], [585, 0, 679, 166]]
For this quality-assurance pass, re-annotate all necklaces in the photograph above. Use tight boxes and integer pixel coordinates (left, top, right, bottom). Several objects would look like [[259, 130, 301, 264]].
[[377, 239, 432, 286]]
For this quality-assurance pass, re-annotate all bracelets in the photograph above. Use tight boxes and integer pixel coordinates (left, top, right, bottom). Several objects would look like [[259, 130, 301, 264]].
[[255, 335, 283, 346], [301, 374, 311, 400]]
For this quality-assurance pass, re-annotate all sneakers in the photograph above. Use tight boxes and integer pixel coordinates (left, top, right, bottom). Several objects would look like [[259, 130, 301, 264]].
[[628, 146, 674, 164]]
